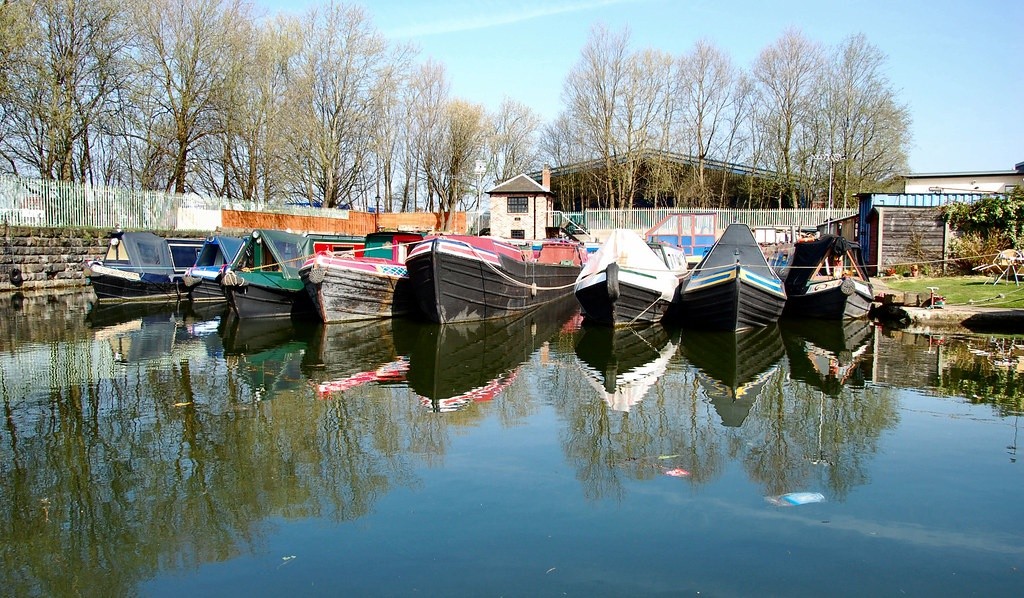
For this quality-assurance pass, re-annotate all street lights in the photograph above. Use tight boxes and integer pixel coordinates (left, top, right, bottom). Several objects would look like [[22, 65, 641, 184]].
[[375, 194, 381, 232]]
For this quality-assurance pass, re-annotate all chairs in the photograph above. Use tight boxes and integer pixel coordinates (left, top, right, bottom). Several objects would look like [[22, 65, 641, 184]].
[[995, 249, 1021, 284], [972, 249, 1005, 286]]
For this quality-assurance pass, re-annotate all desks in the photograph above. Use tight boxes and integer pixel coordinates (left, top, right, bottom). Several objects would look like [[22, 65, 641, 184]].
[[996, 257, 1024, 285]]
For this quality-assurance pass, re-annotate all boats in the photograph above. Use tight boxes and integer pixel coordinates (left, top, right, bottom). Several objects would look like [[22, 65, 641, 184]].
[[82, 231, 206, 301], [646, 240, 692, 284], [216, 303, 316, 359], [84, 302, 225, 341], [767, 233, 873, 321], [181, 235, 250, 300], [405, 230, 586, 325], [297, 228, 424, 324], [571, 318, 680, 413], [679, 328, 788, 402], [780, 330, 877, 400], [574, 229, 682, 325], [300, 318, 411, 400], [391, 295, 582, 414], [679, 223, 787, 334], [219, 229, 367, 320], [184, 300, 227, 337]]
